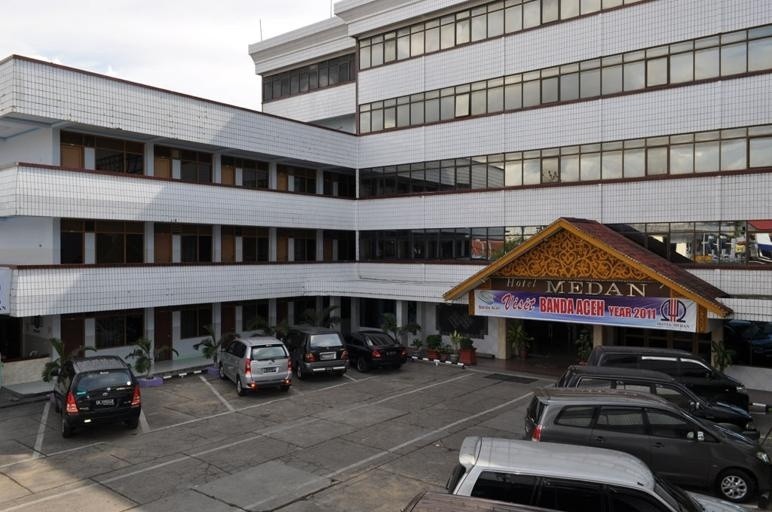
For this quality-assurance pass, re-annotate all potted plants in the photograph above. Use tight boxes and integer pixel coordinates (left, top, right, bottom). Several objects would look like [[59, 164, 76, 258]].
[[410, 328, 479, 368], [192, 320, 243, 380], [120, 337, 180, 388]]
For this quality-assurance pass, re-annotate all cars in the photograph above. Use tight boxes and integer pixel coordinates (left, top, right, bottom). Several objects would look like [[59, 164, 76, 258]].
[[342, 330, 408, 374]]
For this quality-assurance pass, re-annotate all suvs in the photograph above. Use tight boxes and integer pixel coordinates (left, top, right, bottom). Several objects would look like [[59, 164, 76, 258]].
[[445, 434, 759, 512], [553, 364, 753, 430], [277, 324, 349, 381], [587, 344, 750, 411], [53, 355, 141, 439], [218, 333, 293, 397], [521, 385, 772, 506]]
